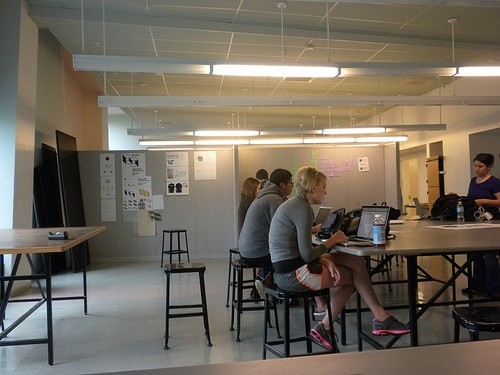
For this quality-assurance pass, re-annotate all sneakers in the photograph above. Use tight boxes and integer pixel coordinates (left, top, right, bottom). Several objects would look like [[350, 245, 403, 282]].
[[309, 322, 338, 351], [372, 312, 410, 336]]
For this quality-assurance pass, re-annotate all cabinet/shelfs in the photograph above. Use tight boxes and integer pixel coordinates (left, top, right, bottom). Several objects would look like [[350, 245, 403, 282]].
[[426, 159, 444, 216]]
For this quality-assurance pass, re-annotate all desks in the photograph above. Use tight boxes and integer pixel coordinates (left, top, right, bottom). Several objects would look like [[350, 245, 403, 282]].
[[312, 221, 500, 352], [0, 226, 106, 366], [83, 339, 500, 375]]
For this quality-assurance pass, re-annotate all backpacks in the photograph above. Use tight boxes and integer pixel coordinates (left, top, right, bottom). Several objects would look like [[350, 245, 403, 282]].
[[316, 208, 395, 240], [430, 194, 478, 221]]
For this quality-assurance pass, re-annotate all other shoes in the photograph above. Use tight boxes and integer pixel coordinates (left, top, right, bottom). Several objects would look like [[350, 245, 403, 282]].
[[255, 276, 267, 299], [461, 288, 475, 293], [313, 310, 327, 315]]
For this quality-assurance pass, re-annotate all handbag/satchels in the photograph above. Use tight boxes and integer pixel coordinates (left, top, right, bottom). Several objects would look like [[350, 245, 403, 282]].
[[373, 201, 401, 220]]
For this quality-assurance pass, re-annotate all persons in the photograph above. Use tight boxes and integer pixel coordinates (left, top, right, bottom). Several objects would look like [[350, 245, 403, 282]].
[[238, 168, 328, 316], [269, 166, 411, 353], [448, 153, 500, 299]]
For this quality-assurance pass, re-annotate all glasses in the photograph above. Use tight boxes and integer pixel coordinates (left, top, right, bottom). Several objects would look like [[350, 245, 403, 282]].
[[289, 181, 294, 186]]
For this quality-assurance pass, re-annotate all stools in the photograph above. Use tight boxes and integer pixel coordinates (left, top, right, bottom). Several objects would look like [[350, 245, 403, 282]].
[[161, 229, 190, 267], [226, 247, 336, 360], [452, 307, 500, 343], [164, 262, 212, 350]]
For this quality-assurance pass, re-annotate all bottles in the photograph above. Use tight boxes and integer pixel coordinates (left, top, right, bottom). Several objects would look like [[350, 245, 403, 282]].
[[457, 201, 466, 222], [372, 214, 386, 248]]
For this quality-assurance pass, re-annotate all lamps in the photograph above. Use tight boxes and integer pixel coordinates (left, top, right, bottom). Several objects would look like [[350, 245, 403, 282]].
[[72, 1, 500, 147]]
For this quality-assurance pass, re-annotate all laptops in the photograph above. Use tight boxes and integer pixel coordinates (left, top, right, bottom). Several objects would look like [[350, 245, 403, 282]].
[[313, 207, 332, 226], [337, 206, 390, 246], [413, 198, 425, 217]]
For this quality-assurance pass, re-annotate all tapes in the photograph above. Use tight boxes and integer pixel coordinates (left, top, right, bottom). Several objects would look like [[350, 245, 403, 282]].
[[56, 232, 61, 235]]
